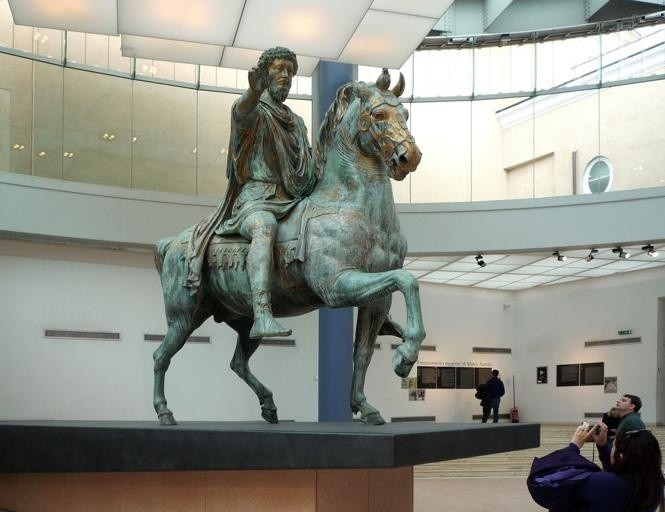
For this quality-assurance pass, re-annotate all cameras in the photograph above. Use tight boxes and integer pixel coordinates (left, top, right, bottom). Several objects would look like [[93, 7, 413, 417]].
[[581, 421, 601, 438]]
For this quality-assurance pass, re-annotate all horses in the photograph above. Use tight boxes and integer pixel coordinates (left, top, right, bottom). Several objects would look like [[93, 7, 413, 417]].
[[150, 66, 427, 426]]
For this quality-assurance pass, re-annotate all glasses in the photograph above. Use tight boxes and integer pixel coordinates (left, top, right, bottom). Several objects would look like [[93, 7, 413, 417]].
[[624, 429, 651, 445]]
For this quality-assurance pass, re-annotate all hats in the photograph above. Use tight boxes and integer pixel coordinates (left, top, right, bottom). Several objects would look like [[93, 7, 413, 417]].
[[493, 369, 498, 376]]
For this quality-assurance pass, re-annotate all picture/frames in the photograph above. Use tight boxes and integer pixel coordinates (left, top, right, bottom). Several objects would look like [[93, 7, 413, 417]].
[[401, 366, 493, 401], [557, 362, 618, 394], [537, 366, 548, 383]]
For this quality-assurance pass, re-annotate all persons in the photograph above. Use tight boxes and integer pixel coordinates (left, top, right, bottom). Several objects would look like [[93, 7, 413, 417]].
[[602, 407, 621, 448], [614, 394, 645, 439], [526, 421, 664, 511], [480, 370, 505, 423], [181, 46, 318, 339]]
